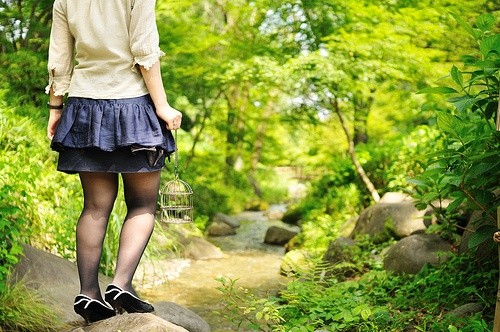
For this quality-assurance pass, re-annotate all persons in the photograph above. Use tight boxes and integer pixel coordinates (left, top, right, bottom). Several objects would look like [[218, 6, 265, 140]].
[[47, 0, 182, 326]]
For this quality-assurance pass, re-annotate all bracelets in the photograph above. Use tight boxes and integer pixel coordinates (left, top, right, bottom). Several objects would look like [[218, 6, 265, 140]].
[[47, 101, 63, 109]]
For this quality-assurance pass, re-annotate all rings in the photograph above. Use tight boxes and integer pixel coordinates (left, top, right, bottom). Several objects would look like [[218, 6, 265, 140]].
[[173, 122, 177, 126]]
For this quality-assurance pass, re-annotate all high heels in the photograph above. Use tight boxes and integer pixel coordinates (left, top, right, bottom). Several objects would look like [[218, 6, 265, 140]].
[[105, 284, 155, 315], [73, 294, 116, 326]]
